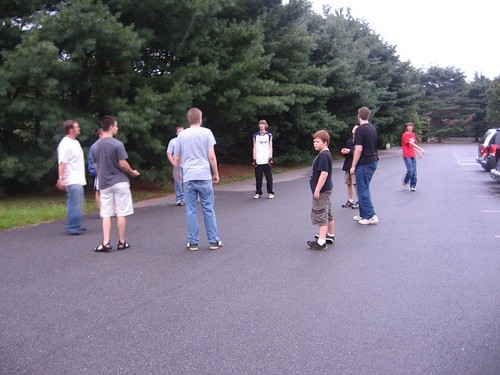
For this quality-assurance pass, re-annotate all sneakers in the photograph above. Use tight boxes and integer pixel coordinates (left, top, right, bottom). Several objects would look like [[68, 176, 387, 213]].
[[352, 201, 360, 209], [359, 215, 379, 225], [342, 199, 354, 207], [210, 241, 224, 249], [187, 242, 199, 250], [353, 215, 362, 220], [315, 233, 335, 244], [307, 240, 327, 250]]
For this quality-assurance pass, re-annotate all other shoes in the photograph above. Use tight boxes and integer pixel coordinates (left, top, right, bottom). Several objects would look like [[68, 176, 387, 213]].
[[66, 230, 83, 235], [411, 186, 416, 191], [268, 194, 275, 199], [80, 227, 86, 232], [176, 199, 185, 206], [254, 194, 261, 198]]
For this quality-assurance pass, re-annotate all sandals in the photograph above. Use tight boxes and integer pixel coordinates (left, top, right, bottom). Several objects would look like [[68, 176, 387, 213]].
[[93, 242, 112, 251], [117, 240, 130, 250]]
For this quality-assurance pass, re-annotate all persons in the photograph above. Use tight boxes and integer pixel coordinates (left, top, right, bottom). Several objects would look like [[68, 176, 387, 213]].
[[167, 125, 184, 205], [401, 122, 425, 191], [88, 129, 103, 218], [57, 120, 86, 234], [350, 107, 379, 224], [306, 130, 335, 249], [174, 108, 224, 250], [253, 120, 274, 199], [91, 116, 140, 252], [341, 125, 359, 209]]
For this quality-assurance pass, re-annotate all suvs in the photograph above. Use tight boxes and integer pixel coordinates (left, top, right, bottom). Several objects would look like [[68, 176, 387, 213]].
[[476, 128, 500, 181]]
